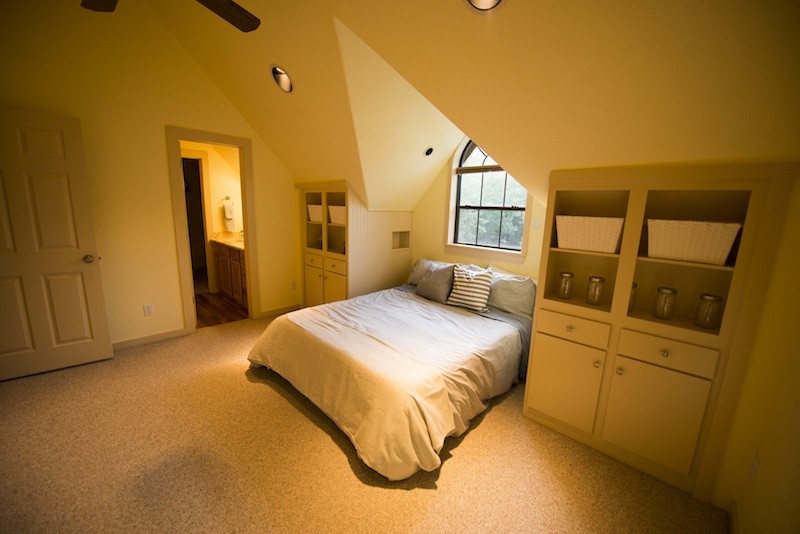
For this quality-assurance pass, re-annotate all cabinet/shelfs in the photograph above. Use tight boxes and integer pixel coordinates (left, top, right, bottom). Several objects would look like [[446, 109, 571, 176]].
[[299, 187, 349, 308], [522, 178, 772, 496], [212, 242, 247, 313]]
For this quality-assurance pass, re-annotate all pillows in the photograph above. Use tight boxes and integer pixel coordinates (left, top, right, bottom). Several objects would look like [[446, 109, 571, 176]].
[[446, 266, 493, 313], [486, 264, 537, 321], [415, 262, 456, 305], [406, 258, 461, 285]]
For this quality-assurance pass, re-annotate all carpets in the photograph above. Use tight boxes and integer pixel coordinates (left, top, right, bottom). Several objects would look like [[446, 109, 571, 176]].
[[196, 293, 248, 328]]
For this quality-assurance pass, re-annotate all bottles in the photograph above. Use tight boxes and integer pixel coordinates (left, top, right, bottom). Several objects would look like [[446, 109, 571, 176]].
[[585, 276, 605, 306], [555, 271, 574, 300], [628, 282, 640, 313], [694, 292, 723, 329], [653, 286, 678, 320]]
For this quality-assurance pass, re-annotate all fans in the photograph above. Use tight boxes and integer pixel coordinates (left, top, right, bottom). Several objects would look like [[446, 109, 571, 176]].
[[80, 0, 261, 32]]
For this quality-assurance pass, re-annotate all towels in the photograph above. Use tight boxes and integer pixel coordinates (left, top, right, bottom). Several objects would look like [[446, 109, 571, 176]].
[[223, 200, 241, 233]]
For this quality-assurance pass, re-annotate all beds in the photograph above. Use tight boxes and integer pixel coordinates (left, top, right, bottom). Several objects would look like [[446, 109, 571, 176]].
[[248, 264, 532, 481]]
